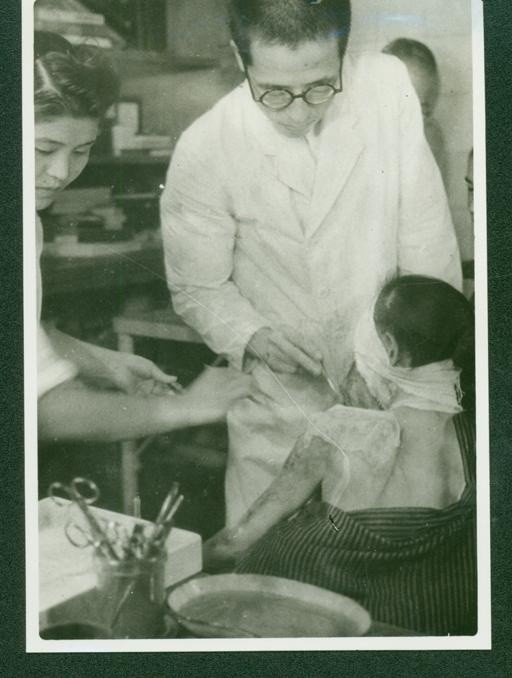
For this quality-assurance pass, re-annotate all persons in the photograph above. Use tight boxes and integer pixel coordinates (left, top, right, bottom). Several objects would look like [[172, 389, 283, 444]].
[[387, 37, 449, 178], [465, 152, 475, 230], [36, 30, 259, 444], [158, 0, 459, 526], [203, 275, 475, 637]]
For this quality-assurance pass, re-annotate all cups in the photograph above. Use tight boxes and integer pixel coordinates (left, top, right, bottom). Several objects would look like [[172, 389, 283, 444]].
[[90, 539, 168, 638]]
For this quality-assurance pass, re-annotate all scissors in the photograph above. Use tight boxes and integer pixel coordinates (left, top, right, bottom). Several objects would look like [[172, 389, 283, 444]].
[[65, 514, 107, 562], [109, 483, 185, 629], [50, 477, 120, 561]]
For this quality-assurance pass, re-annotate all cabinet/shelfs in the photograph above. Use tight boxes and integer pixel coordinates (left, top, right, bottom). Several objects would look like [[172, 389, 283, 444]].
[[36, 42, 219, 338]]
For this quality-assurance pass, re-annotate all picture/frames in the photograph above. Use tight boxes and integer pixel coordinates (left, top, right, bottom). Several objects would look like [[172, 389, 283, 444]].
[[0, 0, 512, 678]]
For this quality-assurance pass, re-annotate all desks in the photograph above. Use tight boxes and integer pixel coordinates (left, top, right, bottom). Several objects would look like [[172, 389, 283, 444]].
[[163, 571, 429, 638], [111, 299, 204, 519]]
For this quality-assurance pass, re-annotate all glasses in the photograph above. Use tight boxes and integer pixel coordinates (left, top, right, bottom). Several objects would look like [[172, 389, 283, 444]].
[[239, 54, 342, 110]]
